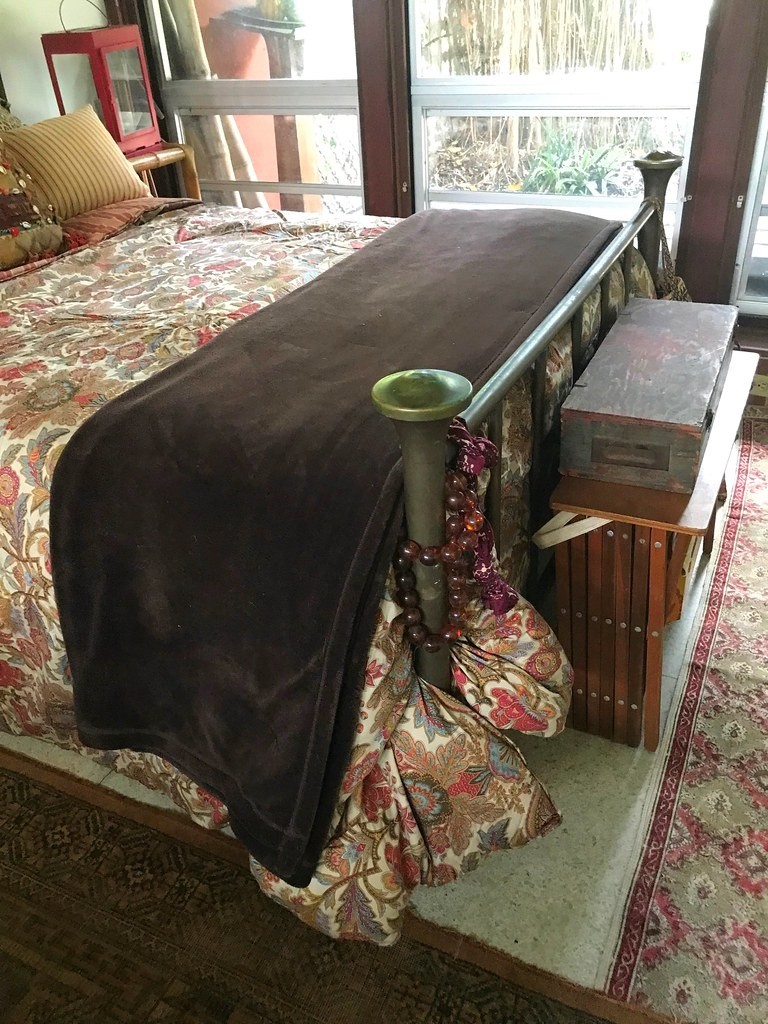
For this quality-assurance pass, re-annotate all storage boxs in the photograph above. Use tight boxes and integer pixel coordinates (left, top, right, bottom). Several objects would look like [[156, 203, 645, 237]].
[[557, 297, 742, 499], [40, 25, 162, 156]]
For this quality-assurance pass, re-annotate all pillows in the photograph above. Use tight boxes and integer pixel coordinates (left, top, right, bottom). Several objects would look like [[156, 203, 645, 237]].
[[0, 104, 149, 221]]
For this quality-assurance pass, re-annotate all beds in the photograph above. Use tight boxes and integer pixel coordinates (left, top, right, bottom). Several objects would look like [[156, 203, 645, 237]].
[[0, 148, 684, 948]]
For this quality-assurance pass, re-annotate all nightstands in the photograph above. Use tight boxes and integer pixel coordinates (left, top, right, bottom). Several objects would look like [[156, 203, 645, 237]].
[[125, 142, 201, 202]]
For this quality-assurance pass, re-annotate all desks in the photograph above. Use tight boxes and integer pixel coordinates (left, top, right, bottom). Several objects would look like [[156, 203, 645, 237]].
[[551, 350, 766, 755]]
